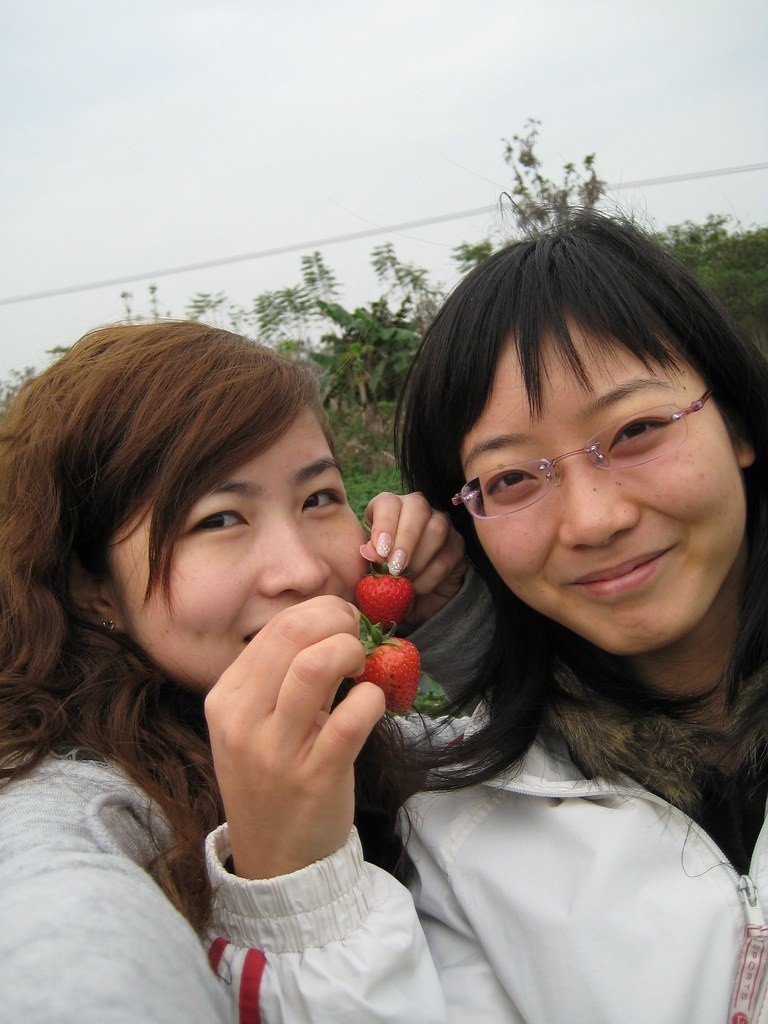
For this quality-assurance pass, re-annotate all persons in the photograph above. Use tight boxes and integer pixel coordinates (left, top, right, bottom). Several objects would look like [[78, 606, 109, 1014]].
[[0, 320, 467, 1024], [203, 203, 768, 1024]]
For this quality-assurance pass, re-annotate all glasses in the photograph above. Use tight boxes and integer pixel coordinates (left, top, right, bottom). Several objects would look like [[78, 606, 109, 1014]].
[[451, 388, 715, 521]]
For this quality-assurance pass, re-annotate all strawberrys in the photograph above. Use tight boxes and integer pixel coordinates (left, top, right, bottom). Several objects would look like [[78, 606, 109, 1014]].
[[356, 562, 420, 714]]
